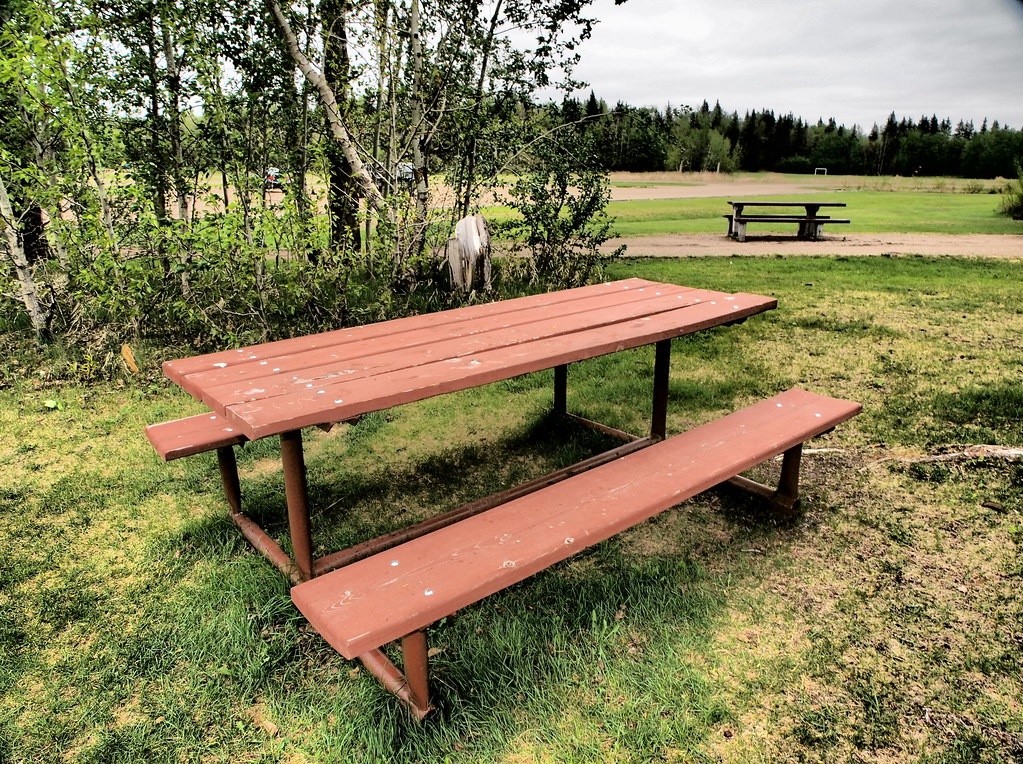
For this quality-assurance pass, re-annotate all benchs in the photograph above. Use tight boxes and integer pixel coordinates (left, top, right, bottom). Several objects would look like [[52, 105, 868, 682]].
[[723, 213, 831, 237], [735, 218, 850, 242], [144, 362, 568, 513], [291, 387, 867, 723]]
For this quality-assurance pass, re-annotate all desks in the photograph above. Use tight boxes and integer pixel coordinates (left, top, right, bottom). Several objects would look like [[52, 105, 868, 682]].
[[727, 200, 846, 241], [161, 278, 779, 583]]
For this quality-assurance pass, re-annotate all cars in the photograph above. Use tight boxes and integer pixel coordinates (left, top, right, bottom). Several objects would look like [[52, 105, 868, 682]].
[[259, 167, 288, 193], [397, 162, 415, 182]]
